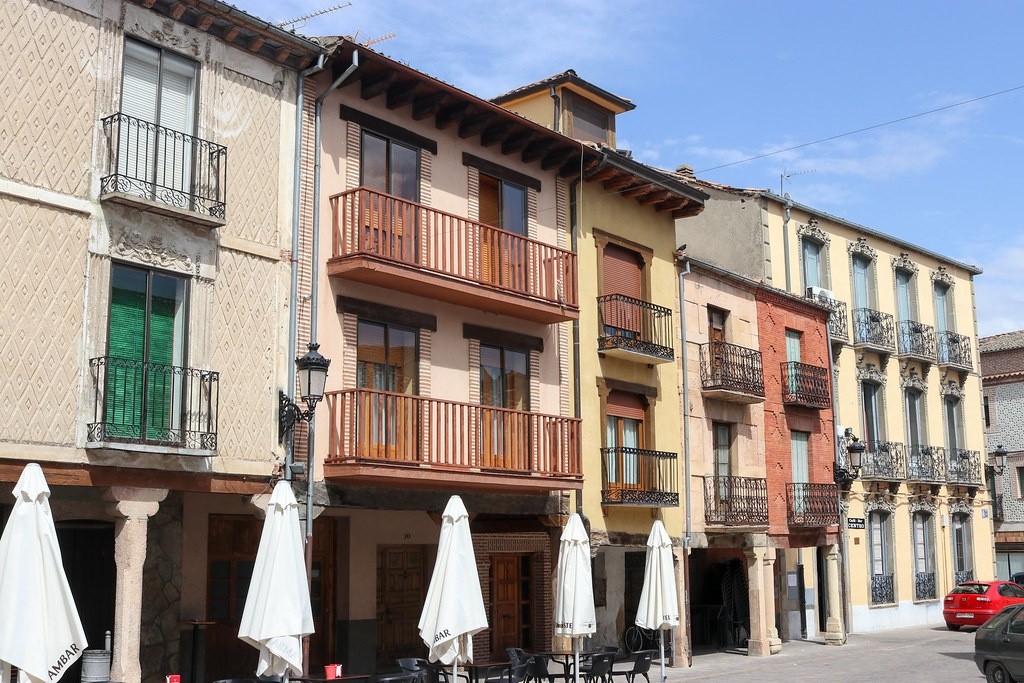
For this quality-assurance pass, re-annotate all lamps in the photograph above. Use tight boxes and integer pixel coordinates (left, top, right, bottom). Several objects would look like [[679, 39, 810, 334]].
[[832, 435, 866, 484], [984, 445, 1007, 479], [278, 342, 332, 444]]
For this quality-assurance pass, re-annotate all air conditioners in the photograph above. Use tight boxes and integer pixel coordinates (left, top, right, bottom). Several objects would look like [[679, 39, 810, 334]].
[[806, 286, 837, 311]]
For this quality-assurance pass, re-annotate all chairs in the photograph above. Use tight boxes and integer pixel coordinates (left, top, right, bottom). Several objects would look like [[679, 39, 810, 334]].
[[214, 643, 659, 683]]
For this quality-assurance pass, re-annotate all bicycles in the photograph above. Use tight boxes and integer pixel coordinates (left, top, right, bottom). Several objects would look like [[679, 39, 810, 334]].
[[625, 614, 673, 653]]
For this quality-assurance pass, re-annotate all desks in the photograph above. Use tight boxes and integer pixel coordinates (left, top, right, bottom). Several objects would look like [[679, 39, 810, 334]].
[[289, 672, 372, 683], [414, 662, 513, 683], [536, 651, 598, 683]]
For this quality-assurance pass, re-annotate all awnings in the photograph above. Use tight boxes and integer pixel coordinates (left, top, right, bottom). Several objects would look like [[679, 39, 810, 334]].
[[268, 477, 572, 517]]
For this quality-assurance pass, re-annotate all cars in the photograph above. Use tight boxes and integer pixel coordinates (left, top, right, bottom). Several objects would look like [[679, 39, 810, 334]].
[[943, 581, 1024, 630], [975, 604, 1024, 683], [1008, 572, 1024, 594]]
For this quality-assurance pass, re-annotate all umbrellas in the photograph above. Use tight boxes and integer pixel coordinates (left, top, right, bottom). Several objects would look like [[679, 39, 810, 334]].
[[417, 493, 490, 683], [633, 519, 681, 683], [0, 461, 91, 683], [551, 510, 598, 683], [236, 478, 318, 683]]
